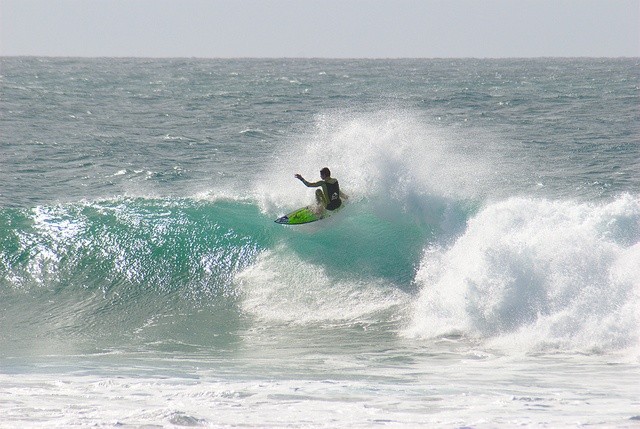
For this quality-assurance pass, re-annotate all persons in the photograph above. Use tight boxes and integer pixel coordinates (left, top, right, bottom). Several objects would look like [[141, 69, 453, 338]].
[[294, 167, 349, 210]]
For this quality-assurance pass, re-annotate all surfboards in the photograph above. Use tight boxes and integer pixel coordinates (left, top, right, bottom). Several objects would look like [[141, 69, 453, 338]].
[[274, 195, 349, 225]]
